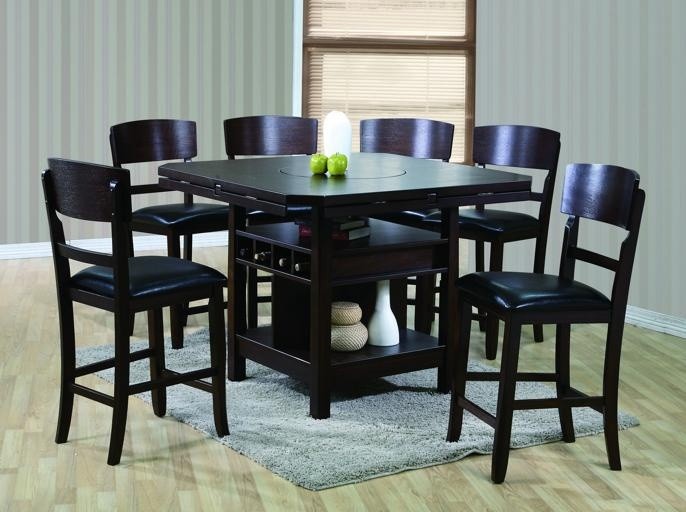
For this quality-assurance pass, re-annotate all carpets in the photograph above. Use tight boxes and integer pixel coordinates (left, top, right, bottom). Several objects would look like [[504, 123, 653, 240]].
[[75, 317, 640, 491]]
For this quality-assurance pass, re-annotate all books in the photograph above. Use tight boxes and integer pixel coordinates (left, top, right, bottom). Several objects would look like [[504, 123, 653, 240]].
[[295, 217, 371, 242]]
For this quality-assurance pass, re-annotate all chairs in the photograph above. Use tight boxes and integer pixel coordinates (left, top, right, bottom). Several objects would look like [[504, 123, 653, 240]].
[[426, 124, 561, 360], [110, 118, 230, 349], [224, 114, 319, 303], [42, 157, 230, 467], [359, 116, 455, 330], [446, 163, 645, 481]]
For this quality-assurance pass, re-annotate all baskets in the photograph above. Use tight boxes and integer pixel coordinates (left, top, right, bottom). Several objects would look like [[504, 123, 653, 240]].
[[330, 301, 368, 352]]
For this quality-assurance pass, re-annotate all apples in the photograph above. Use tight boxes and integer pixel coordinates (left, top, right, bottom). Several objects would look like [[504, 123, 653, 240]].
[[310, 152, 347, 176]]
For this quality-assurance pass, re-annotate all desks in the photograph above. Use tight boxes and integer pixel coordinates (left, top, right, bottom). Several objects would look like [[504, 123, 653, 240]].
[[157, 153, 533, 420]]
